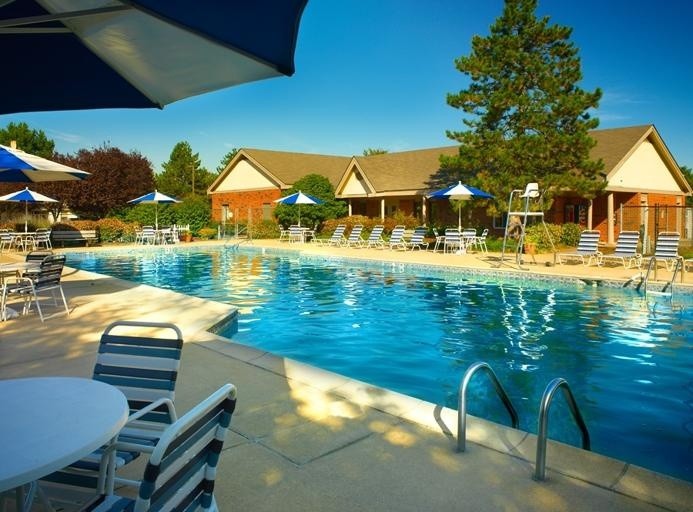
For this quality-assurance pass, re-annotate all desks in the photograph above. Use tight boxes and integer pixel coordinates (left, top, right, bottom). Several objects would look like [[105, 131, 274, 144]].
[[1, 376, 127, 511]]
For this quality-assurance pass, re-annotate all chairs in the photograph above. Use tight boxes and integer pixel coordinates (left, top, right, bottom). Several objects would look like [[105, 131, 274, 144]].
[[21, 321, 183, 511], [519, 184, 539, 212], [134, 225, 179, 244], [0, 227, 52, 253], [0, 249, 71, 323], [59, 381, 234, 511], [309, 225, 491, 259], [278, 225, 317, 243], [556, 228, 693, 274]]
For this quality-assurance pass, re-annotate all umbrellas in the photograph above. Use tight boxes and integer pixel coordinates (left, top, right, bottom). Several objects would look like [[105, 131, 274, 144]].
[[0, 143, 93, 183], [0, 0, 308, 118], [425, 180, 496, 234], [273, 189, 325, 228], [0, 187, 60, 233], [126, 188, 184, 232]]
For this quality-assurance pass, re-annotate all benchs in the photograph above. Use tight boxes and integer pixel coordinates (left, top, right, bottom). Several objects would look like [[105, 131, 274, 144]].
[[50, 229, 98, 247]]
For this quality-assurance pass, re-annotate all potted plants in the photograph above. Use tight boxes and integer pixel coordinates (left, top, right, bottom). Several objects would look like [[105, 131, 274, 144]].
[[522, 226, 541, 255], [185, 232, 191, 242]]
